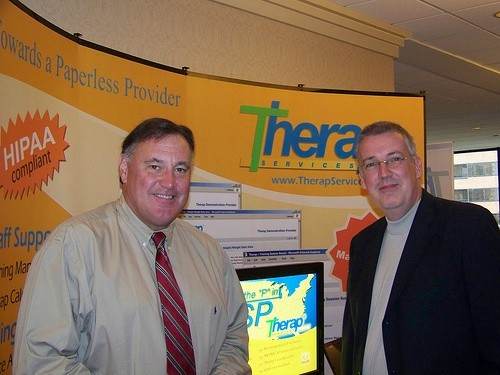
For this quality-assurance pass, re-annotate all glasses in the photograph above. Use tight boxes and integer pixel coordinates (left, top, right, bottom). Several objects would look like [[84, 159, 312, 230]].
[[363, 153, 417, 169]]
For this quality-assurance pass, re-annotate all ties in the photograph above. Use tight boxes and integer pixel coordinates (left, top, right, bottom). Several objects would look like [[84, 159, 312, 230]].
[[151, 231, 197, 375]]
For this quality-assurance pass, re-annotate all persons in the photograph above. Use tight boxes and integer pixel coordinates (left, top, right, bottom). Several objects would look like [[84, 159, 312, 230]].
[[12, 118, 253, 375], [339, 121, 500, 375]]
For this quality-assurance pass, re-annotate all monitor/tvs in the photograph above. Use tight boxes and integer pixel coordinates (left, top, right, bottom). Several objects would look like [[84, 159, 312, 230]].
[[233, 262, 325, 375]]
